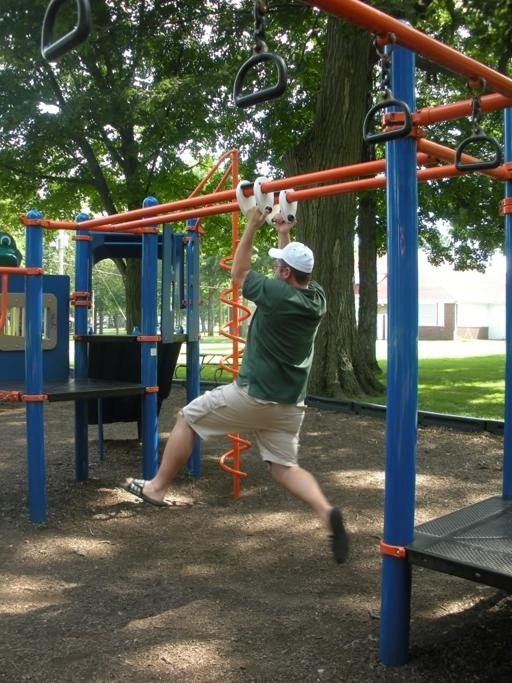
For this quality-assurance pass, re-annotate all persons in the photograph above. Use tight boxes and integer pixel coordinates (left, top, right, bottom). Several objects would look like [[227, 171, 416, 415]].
[[119, 205, 348, 563]]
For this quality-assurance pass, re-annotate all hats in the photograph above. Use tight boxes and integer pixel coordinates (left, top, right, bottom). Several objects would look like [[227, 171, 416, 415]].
[[268, 242, 314, 273]]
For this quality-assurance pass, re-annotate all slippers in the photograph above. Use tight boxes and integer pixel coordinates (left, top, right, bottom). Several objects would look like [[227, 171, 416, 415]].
[[118, 477, 163, 507], [326, 507, 348, 564]]
